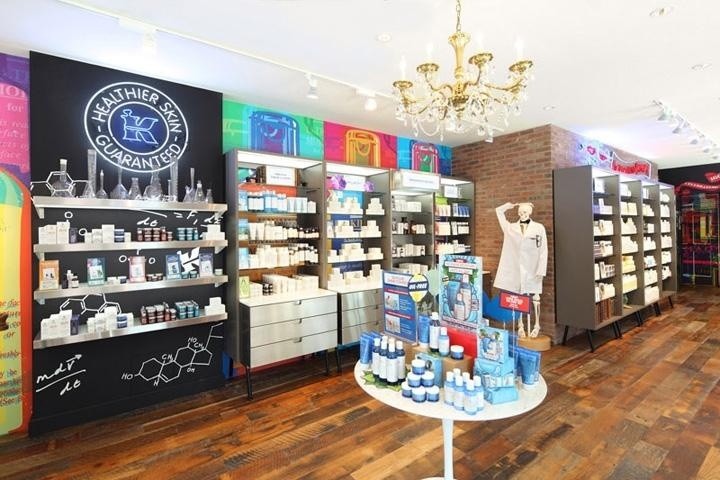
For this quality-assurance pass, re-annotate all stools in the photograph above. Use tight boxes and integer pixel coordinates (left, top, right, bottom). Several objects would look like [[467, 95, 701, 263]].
[[517, 332, 552, 352]]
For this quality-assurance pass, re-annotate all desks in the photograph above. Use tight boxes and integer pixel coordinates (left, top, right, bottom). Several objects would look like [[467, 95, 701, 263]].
[[354, 357, 548, 480]]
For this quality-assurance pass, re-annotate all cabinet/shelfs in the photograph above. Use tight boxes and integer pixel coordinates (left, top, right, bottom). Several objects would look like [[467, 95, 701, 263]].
[[225, 148, 339, 400], [29, 195, 228, 435], [324, 163, 391, 371], [435, 176, 491, 296], [391, 170, 441, 276], [553, 165, 678, 353]]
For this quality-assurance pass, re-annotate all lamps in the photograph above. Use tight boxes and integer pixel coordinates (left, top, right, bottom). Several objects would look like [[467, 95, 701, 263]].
[[391, 1, 532, 142]]
